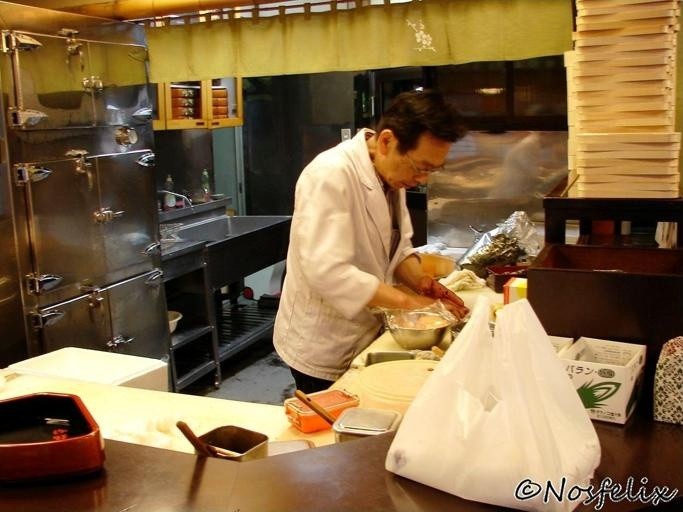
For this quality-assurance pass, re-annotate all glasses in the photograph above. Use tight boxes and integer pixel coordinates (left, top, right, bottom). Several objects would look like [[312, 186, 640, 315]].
[[405, 153, 446, 178]]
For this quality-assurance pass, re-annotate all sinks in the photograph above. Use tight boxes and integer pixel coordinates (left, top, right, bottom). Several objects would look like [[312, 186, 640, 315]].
[[172, 215, 293, 289]]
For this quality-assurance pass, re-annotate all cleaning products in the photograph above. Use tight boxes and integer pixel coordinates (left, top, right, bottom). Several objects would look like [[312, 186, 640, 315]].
[[200, 168, 210, 202], [162, 174, 178, 208]]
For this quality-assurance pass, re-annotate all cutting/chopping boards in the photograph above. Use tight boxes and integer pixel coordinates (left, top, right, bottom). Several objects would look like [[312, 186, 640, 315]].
[[358, 359, 439, 400]]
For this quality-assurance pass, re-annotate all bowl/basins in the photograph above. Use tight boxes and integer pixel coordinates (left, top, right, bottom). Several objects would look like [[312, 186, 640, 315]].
[[384, 311, 452, 349], [167, 312, 184, 334]]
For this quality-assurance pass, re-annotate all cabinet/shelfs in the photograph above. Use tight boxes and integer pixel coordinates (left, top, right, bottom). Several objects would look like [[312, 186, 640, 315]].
[[165, 76, 244, 131]]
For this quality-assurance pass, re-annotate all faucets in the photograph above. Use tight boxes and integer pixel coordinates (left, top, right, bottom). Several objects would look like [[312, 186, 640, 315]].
[[157, 189, 196, 213]]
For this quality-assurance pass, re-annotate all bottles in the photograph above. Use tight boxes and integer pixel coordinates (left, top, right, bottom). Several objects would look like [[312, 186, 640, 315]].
[[165, 174, 176, 210], [201, 168, 210, 198]]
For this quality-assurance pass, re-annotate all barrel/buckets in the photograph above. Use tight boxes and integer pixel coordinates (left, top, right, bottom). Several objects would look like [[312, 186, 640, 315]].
[[244, 259, 286, 301]]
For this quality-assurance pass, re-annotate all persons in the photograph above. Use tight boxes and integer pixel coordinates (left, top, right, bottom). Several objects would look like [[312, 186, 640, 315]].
[[272, 89, 470, 393], [490, 128, 560, 198]]
